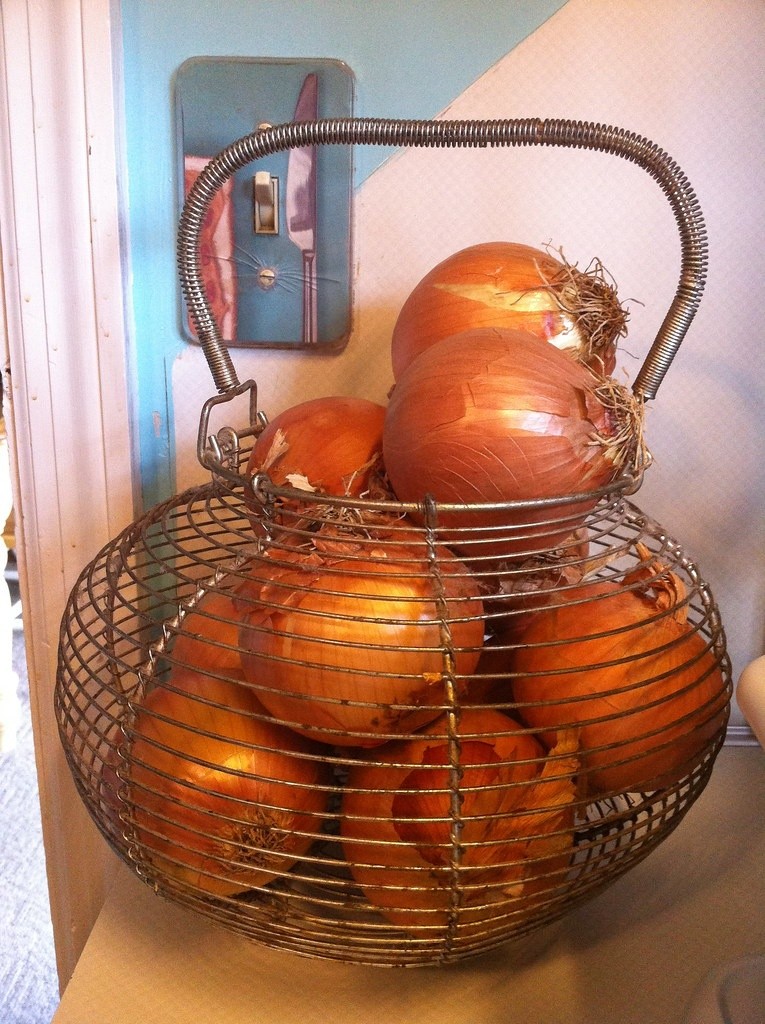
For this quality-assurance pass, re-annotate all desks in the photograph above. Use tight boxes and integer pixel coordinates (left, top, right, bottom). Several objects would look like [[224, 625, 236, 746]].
[[47, 750, 765, 1024]]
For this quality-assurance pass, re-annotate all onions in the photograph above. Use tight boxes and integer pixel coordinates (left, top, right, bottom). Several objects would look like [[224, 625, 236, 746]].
[[103, 244, 726, 955]]
